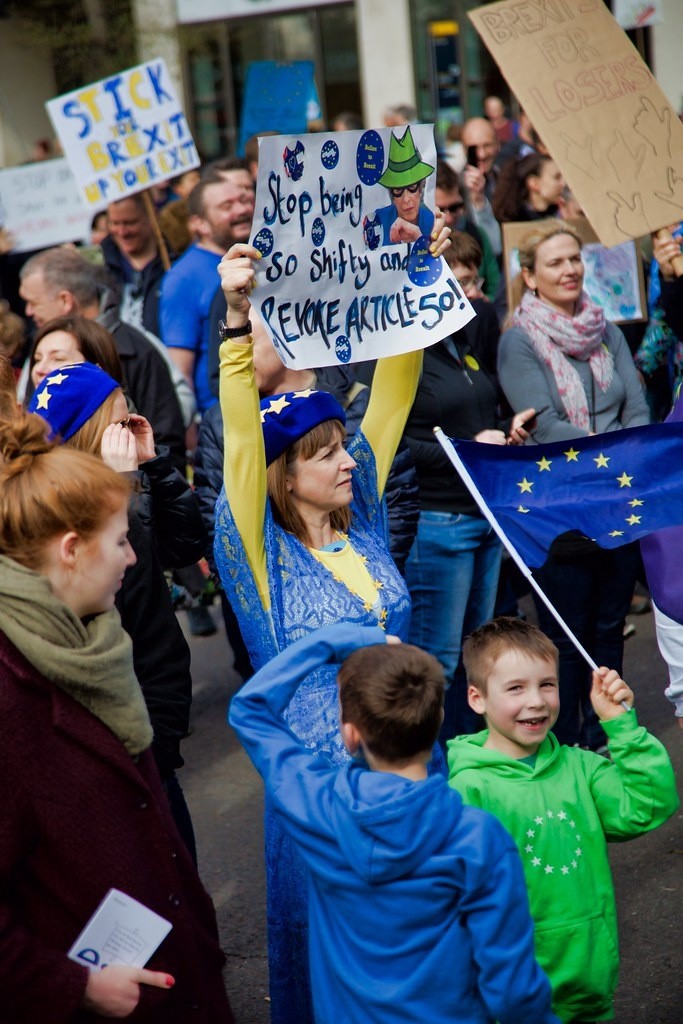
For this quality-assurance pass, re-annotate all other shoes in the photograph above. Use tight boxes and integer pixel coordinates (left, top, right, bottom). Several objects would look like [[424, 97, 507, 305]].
[[630, 595, 649, 613], [622, 623, 636, 637], [187, 604, 216, 636]]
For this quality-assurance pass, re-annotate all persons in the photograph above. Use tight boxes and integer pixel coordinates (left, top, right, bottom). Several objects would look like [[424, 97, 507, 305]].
[[212, 205, 451, 1024], [0, 409, 226, 1024], [374, 179, 435, 246], [446, 617, 681, 1024], [227, 624, 559, 1024], [25, 362, 209, 871], [0, 96, 683, 770]]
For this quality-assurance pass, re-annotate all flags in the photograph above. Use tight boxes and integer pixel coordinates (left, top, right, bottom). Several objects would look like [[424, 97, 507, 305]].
[[450, 421, 683, 568]]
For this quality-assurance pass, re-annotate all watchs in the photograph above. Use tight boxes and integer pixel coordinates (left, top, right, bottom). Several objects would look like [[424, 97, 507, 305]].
[[218, 320, 252, 341]]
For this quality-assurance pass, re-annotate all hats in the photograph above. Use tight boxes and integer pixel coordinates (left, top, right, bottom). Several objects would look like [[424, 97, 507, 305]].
[[28, 361, 121, 445], [259, 389, 345, 469]]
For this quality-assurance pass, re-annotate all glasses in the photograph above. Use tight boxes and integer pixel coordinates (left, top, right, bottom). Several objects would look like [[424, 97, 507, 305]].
[[440, 199, 462, 214], [119, 417, 132, 430]]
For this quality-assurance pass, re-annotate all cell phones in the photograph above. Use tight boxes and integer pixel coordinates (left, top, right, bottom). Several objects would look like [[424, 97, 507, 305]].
[[516, 406, 550, 432], [465, 145, 479, 168]]
